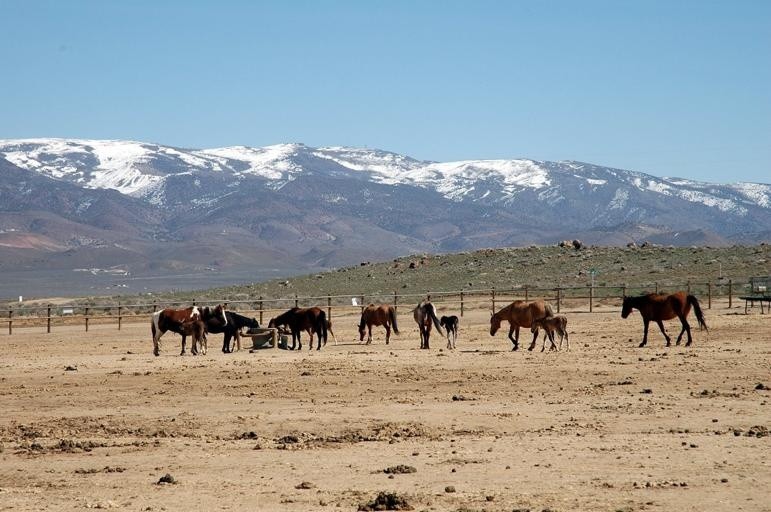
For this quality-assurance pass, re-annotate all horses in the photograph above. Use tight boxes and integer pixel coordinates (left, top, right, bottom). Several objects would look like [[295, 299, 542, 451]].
[[150, 288, 711, 356]]
